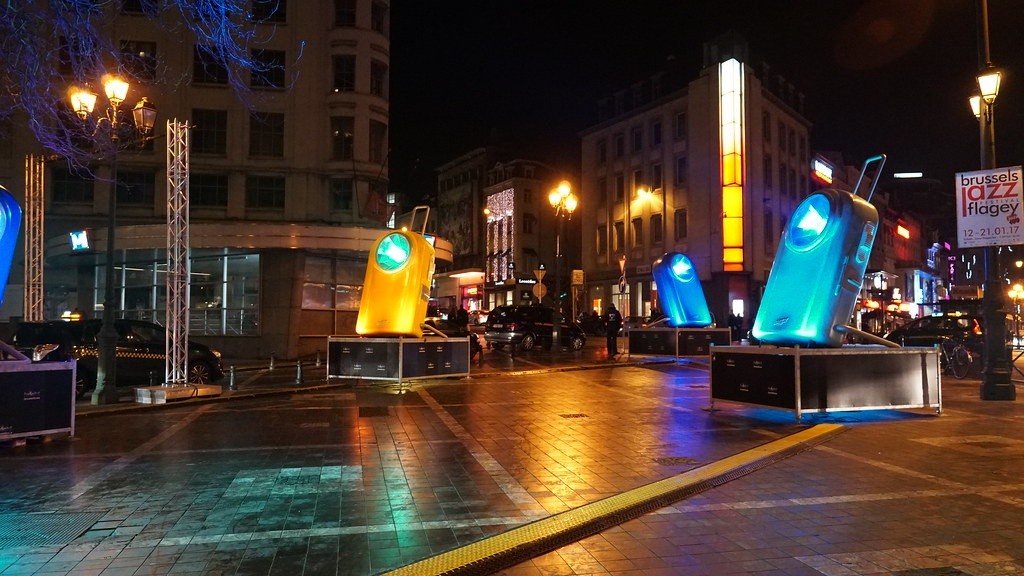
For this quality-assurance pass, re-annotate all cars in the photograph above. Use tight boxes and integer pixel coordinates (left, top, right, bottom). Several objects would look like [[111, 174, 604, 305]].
[[468, 311, 489, 325], [436, 308, 451, 320], [621, 316, 652, 335], [1011, 330, 1023, 346], [576, 311, 607, 336], [885, 312, 986, 366], [234, 311, 257, 328], [359, 319, 482, 361]]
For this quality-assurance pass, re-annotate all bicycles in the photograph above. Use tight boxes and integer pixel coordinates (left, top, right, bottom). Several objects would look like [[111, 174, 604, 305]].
[[938, 336, 973, 380]]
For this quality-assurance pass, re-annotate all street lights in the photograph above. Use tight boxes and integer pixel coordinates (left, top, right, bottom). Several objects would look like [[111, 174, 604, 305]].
[[549, 182, 578, 359], [1015, 257, 1024, 350], [969, 63, 1018, 401], [70, 76, 156, 400], [1008, 283, 1024, 348]]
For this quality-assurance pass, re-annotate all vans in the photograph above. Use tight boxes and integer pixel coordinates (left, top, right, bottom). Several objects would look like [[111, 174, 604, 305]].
[[485, 303, 587, 352], [1, 321, 225, 396], [644, 311, 716, 341]]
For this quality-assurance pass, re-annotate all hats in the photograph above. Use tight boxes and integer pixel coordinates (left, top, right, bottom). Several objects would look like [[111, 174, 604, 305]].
[[608, 303, 614, 307]]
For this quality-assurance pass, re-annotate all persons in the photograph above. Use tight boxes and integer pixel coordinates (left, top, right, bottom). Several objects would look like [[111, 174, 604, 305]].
[[601, 303, 622, 354], [457, 305, 468, 327], [448, 304, 456, 320]]
